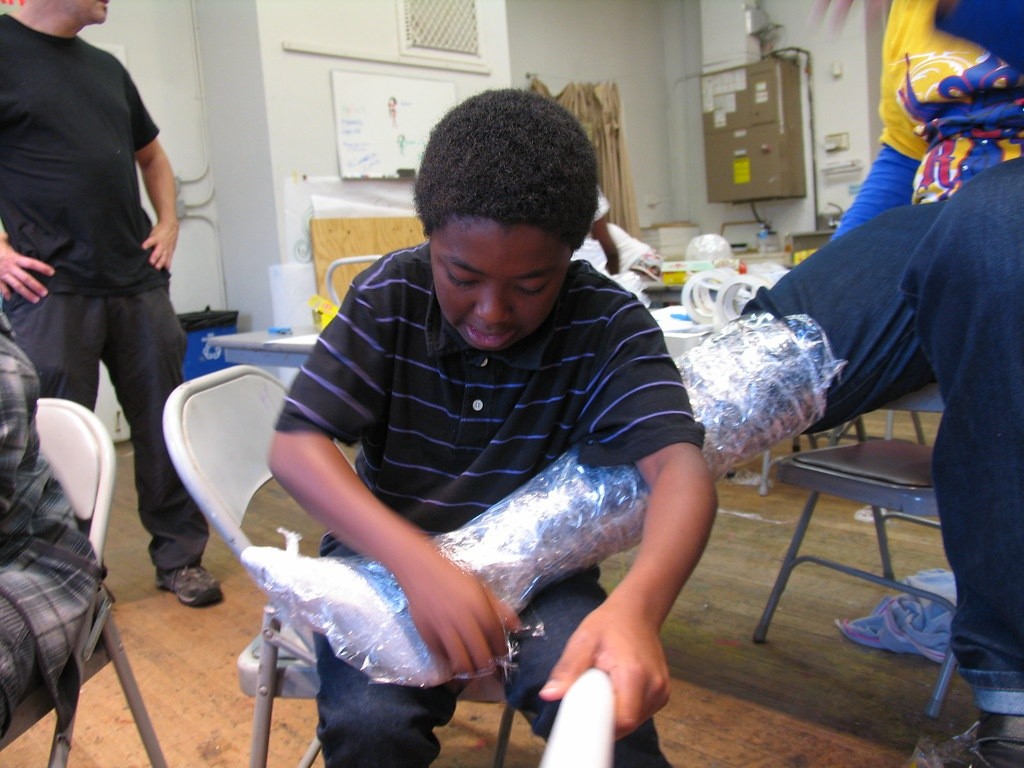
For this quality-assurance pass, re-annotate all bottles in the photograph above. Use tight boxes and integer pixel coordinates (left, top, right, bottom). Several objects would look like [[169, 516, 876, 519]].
[[757, 224, 768, 253]]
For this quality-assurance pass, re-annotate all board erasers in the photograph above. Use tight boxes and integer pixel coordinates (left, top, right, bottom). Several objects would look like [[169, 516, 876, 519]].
[[397, 169, 416, 180]]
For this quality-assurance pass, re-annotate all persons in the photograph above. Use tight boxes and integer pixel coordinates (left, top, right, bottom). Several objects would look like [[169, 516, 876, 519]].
[[243, 154, 1024, 768], [266, 86, 724, 768], [241, 153, 1024, 768], [827, 1, 1024, 241], [1, 1, 226, 608], [0, 312, 102, 739], [592, 182, 620, 275]]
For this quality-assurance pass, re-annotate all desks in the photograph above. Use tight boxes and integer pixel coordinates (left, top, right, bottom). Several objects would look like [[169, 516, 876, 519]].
[[201, 326, 319, 367]]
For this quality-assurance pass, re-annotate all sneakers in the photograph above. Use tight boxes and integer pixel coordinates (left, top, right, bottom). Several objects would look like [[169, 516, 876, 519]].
[[155, 565, 222, 607]]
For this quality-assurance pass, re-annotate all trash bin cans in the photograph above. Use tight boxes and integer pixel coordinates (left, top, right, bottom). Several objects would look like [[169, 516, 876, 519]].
[[178, 310, 238, 380]]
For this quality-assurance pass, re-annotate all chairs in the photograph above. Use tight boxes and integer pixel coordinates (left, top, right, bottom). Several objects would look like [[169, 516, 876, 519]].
[[750, 383, 958, 722], [0, 396, 167, 768], [163, 365, 515, 768]]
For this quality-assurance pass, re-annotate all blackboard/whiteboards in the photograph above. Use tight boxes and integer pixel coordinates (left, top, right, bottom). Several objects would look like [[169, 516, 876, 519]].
[[330, 69, 457, 179]]
